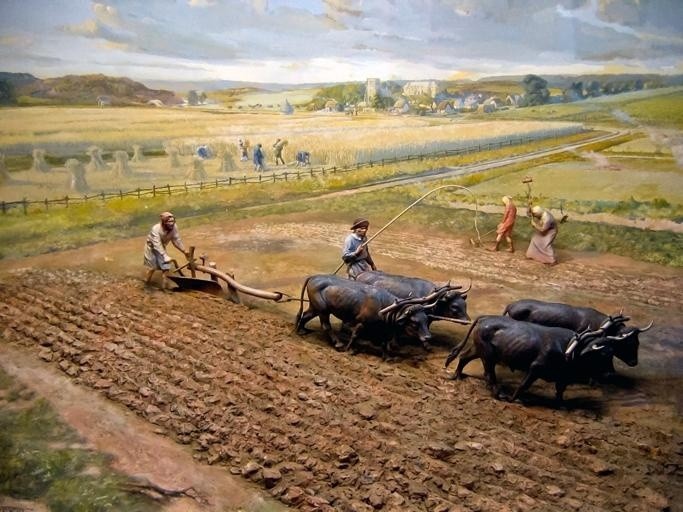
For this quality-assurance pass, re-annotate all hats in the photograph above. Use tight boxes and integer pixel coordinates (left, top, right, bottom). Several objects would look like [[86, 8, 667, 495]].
[[350, 218, 368, 229], [161, 212, 174, 226]]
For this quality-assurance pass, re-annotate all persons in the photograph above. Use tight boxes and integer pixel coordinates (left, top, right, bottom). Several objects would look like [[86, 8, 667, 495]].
[[342, 217, 377, 280], [487, 195, 517, 253], [526, 206, 558, 266], [238, 138, 311, 172], [142, 212, 190, 289]]
